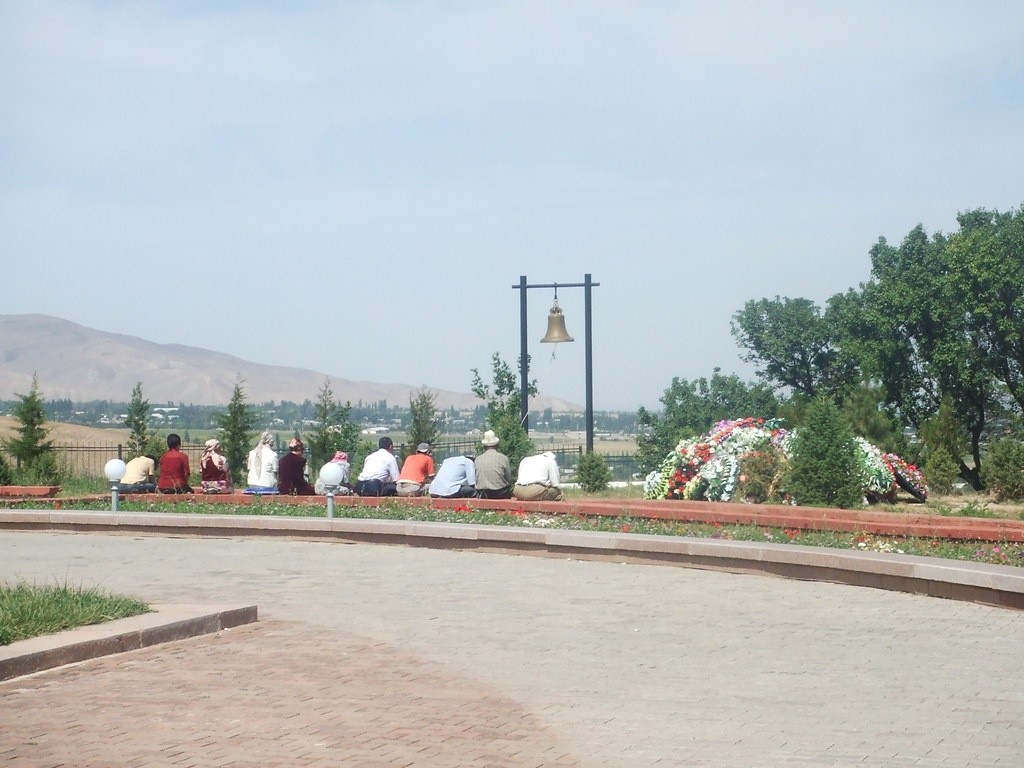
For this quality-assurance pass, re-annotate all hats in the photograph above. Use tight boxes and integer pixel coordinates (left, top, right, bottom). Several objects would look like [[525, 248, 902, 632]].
[[416, 442, 430, 452], [542, 451, 556, 460], [482, 430, 499, 446], [330, 451, 349, 463]]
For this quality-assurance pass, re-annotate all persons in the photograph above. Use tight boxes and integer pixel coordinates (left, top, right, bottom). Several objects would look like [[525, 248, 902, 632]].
[[199, 439, 233, 494], [247, 432, 278, 488], [513, 451, 565, 501], [158, 434, 195, 494], [117, 454, 158, 494], [429, 455, 476, 498], [314, 451, 354, 496], [277, 437, 316, 495], [396, 443, 436, 496], [355, 437, 400, 496], [474, 430, 511, 499]]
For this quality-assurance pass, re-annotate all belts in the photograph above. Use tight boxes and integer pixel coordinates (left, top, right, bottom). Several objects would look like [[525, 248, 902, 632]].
[[369, 479, 378, 482], [517, 482, 539, 486]]
